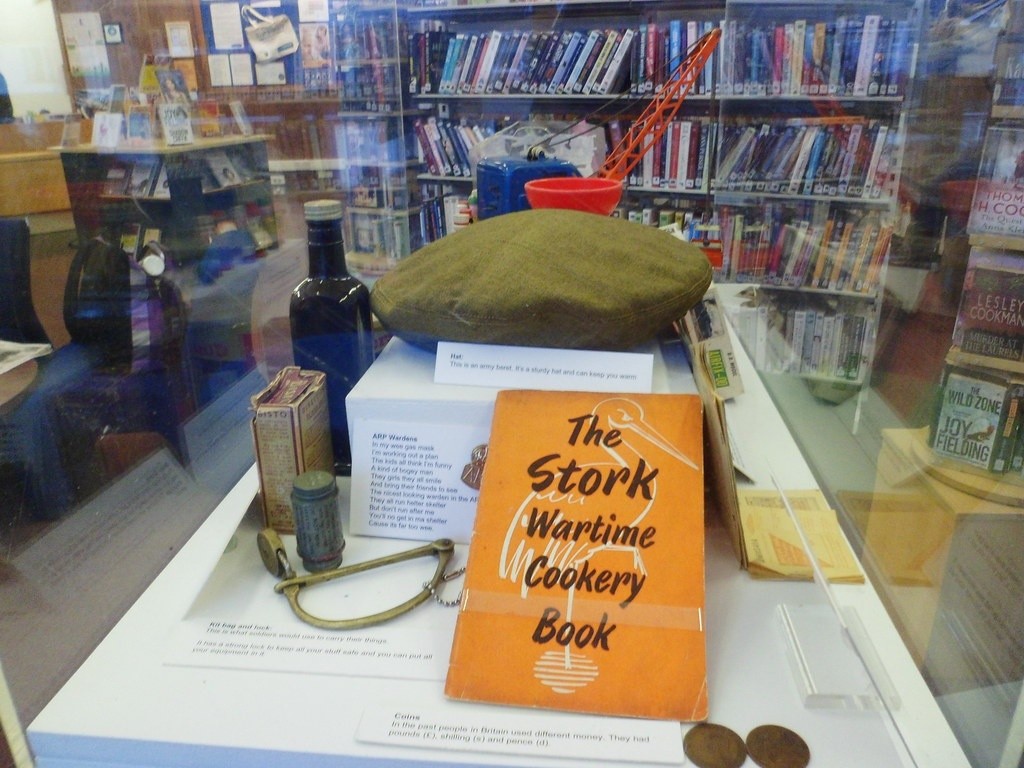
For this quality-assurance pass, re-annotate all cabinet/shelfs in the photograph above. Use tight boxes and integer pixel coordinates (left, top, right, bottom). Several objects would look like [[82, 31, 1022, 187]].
[[913, 3, 1024, 504], [50, 135, 284, 415], [215, 96, 350, 206], [327, 0, 923, 434], [856, 425, 1024, 766]]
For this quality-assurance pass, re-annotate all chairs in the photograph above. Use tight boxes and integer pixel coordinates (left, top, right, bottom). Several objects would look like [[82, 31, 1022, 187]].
[[1, 236, 197, 520], [1, 219, 54, 365]]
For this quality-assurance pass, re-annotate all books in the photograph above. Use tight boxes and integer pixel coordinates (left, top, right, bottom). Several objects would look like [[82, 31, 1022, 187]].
[[59, 53, 272, 270], [932, 1, 1024, 477], [0, 339, 53, 376], [441, 387, 710, 723], [327, 1, 920, 382]]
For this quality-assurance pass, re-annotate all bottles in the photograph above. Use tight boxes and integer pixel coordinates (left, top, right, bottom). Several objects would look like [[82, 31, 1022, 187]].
[[287, 199, 376, 478]]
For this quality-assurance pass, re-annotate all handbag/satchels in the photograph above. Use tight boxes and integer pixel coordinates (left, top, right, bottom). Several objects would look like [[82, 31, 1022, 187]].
[[241, 4, 300, 64]]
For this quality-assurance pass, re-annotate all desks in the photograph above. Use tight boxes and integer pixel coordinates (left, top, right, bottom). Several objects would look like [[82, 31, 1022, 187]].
[[0, 356, 37, 419], [1, 285, 974, 765]]
